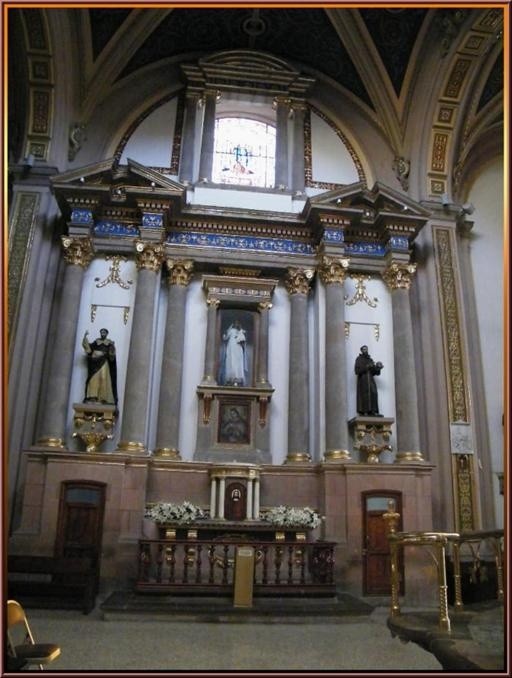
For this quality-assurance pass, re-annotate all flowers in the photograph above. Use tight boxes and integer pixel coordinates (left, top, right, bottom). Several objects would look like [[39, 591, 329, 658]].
[[146, 499, 207, 528], [259, 503, 327, 531]]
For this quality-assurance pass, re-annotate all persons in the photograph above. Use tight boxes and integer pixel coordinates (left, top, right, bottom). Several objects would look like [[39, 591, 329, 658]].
[[79, 327, 119, 405], [218, 318, 248, 388], [222, 407, 248, 445], [231, 490, 242, 502], [353, 344, 386, 418]]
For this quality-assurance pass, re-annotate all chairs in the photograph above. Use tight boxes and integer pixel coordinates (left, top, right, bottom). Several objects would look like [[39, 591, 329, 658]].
[[2, 599, 61, 672]]
